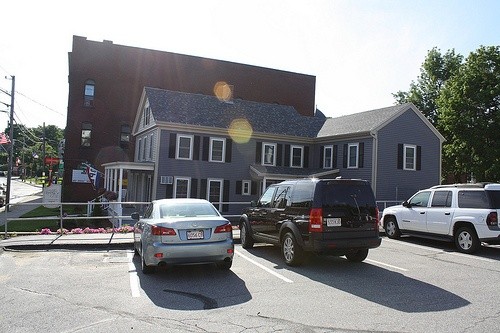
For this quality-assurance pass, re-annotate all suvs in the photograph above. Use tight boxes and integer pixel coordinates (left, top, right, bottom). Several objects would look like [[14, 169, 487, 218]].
[[381, 180, 500, 253], [239, 175, 381, 267]]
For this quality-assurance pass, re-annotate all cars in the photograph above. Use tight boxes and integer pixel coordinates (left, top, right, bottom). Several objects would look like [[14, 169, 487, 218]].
[[0, 182, 7, 208], [130, 197, 236, 275]]
